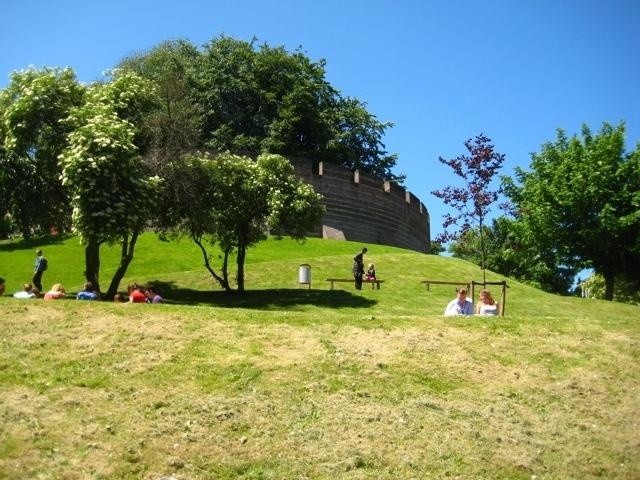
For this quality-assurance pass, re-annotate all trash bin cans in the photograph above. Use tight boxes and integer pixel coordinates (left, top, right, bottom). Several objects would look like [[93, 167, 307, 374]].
[[298, 264, 311, 284]]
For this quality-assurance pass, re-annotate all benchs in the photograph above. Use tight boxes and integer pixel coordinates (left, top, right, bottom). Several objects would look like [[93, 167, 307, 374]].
[[326, 279, 385, 290], [421, 281, 468, 291]]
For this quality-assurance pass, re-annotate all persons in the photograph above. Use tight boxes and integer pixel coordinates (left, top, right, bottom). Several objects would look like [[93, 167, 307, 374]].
[[477, 290, 500, 316], [0, 277, 165, 304], [444, 289, 474, 315], [32, 249, 47, 292], [365, 263, 376, 282], [352, 248, 367, 288]]
